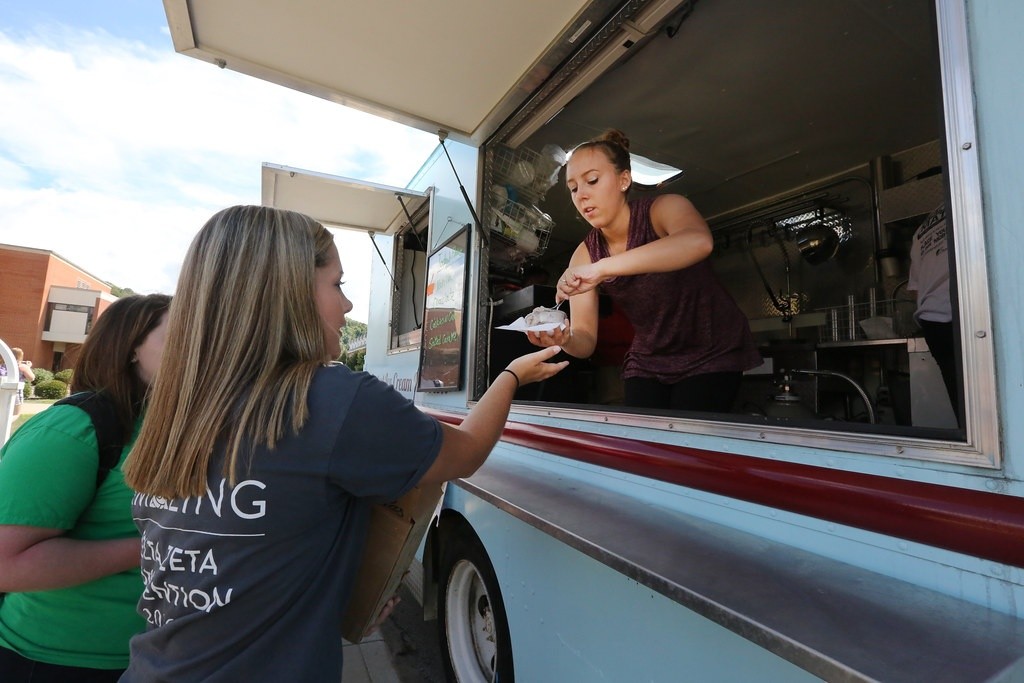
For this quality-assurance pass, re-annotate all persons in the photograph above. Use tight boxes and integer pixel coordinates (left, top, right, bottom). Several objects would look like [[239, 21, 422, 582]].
[[906, 148, 967, 436], [521, 126, 754, 409], [0, 293, 177, 683], [113, 205, 573, 683], [1, 348, 36, 424]]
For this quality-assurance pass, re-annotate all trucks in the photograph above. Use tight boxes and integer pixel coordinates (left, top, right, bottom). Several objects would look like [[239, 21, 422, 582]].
[[163, 1, 1023, 682]]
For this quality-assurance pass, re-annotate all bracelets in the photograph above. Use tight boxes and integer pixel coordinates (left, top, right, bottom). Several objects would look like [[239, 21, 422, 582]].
[[500, 369, 521, 390]]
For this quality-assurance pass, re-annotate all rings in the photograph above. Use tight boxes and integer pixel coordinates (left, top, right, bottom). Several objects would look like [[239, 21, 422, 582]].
[[560, 279, 567, 283]]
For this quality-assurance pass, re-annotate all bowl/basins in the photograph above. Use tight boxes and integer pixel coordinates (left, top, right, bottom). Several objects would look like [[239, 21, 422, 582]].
[[524, 310, 566, 327]]
